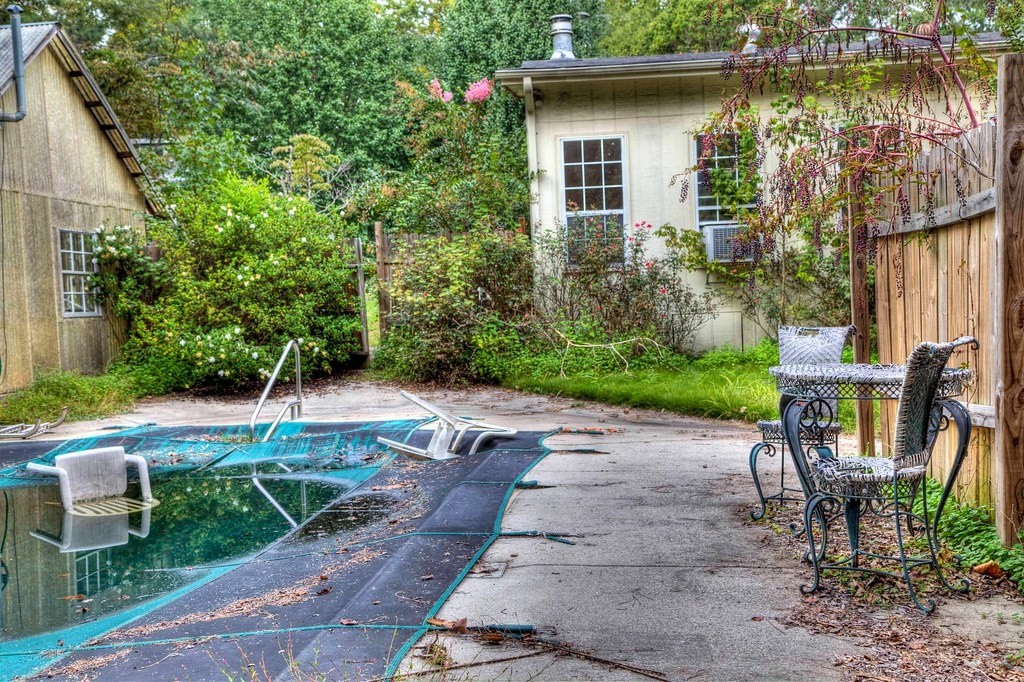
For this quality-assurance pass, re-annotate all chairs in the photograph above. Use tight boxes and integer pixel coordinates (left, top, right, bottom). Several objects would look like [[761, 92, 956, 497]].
[[749, 323, 860, 520], [376, 390, 518, 462], [799, 335, 980, 615], [27, 503, 161, 553], [25, 445, 161, 517]]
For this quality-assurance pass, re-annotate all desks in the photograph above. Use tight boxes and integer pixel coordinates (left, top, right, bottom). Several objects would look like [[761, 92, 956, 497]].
[[770, 363, 974, 565]]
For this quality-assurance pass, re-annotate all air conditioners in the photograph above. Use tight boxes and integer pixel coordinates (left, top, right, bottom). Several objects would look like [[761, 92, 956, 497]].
[[704, 225, 780, 264]]
